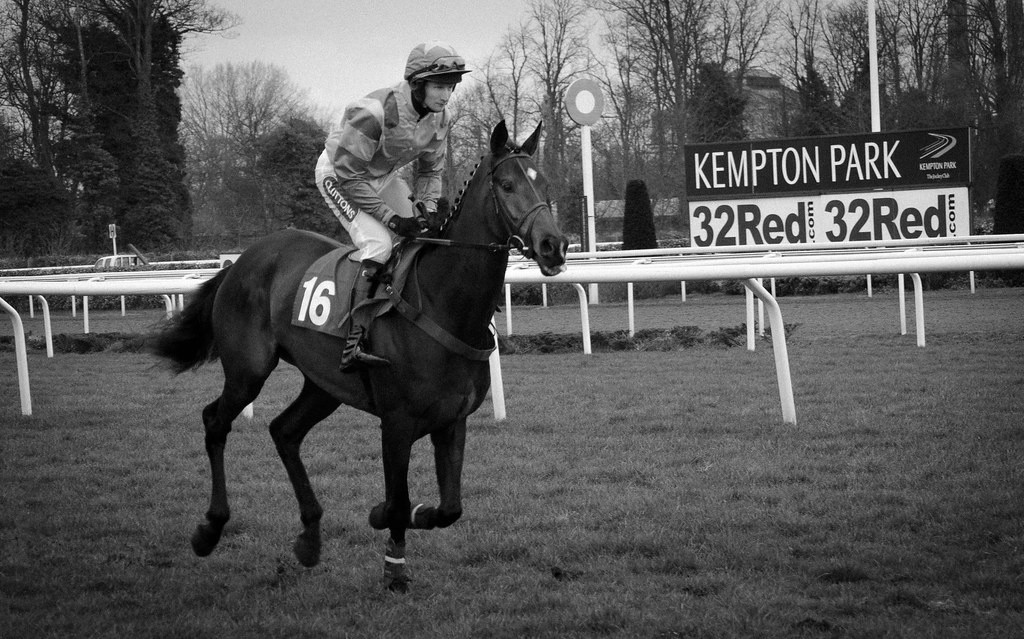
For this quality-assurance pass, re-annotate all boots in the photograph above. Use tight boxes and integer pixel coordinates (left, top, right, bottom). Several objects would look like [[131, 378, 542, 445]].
[[337, 258, 390, 370]]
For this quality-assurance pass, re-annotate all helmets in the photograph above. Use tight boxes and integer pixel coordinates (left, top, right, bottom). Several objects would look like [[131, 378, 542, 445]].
[[404, 41, 471, 84]]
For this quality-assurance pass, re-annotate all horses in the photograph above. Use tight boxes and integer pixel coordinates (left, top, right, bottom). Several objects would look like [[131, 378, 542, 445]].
[[144, 118, 568, 593]]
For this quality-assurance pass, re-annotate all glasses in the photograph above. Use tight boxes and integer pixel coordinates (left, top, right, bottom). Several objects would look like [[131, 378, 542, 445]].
[[430, 54, 466, 73]]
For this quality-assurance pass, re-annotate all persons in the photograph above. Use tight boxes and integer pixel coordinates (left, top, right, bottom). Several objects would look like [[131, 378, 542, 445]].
[[315, 43, 464, 372]]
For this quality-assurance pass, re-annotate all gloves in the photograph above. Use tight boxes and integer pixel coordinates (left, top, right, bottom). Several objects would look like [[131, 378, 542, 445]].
[[387, 215, 420, 239]]
